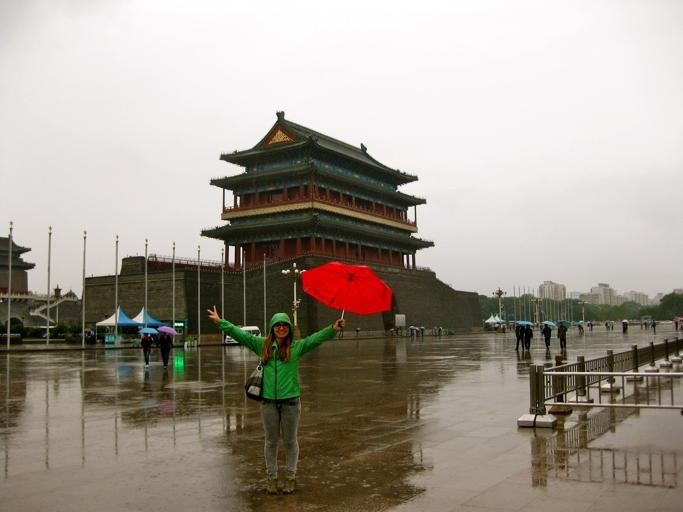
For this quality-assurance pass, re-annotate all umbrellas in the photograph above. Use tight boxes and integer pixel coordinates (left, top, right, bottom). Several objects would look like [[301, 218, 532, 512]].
[[138, 328, 159, 334], [302, 261, 392, 318], [157, 326, 177, 335], [515, 320, 571, 327]]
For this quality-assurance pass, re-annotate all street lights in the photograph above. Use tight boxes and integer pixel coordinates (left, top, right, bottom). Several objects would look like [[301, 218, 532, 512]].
[[578, 300, 588, 321], [492, 288, 507, 324], [53, 284, 62, 324], [281, 263, 307, 327], [531, 297, 542, 323]]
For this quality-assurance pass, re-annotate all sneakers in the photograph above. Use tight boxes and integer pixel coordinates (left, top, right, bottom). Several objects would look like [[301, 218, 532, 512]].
[[280, 476, 297, 493], [266, 477, 280, 495]]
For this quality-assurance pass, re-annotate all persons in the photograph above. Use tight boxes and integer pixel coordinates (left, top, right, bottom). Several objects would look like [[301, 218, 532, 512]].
[[85, 331, 91, 345], [390, 326, 442, 336], [515, 323, 567, 350], [141, 333, 154, 368], [207, 305, 345, 494], [339, 329, 343, 338], [578, 319, 656, 334], [676, 321, 678, 331], [156, 333, 173, 369]]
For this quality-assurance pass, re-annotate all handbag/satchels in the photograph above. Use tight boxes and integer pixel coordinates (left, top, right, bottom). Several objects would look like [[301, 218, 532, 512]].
[[244, 362, 264, 400]]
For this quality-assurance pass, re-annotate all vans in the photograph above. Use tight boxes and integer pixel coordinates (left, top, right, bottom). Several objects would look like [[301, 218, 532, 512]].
[[224, 326, 261, 345]]
